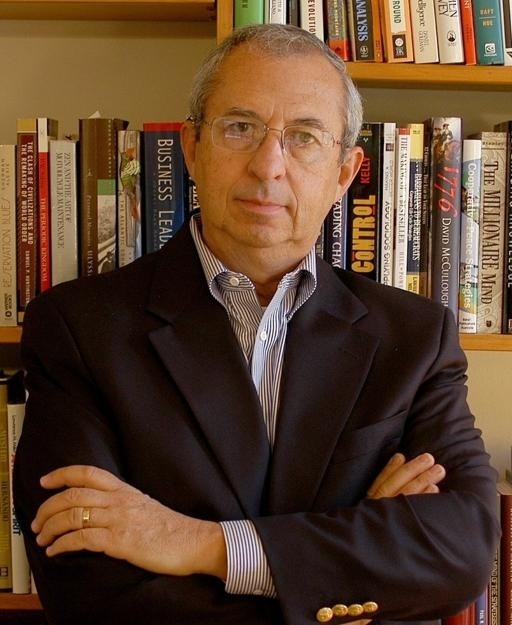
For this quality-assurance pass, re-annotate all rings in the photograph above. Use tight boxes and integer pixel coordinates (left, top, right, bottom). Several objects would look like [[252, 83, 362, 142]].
[[82, 506, 92, 528]]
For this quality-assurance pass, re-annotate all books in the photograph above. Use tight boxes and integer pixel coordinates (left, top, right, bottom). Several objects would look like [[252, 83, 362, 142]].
[[1, 112, 512, 338], [427, 481, 510, 625], [1, 367, 41, 597], [231, 0, 511, 67]]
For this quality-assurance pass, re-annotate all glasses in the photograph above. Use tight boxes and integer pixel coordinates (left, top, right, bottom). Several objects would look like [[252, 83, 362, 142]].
[[187, 117, 351, 161]]
